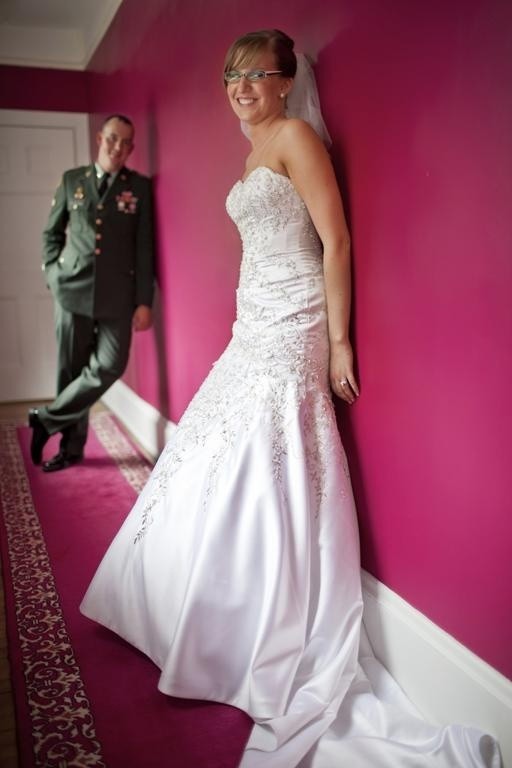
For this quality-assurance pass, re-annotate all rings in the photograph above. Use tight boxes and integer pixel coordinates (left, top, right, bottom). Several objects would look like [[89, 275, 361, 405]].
[[339, 381, 347, 386]]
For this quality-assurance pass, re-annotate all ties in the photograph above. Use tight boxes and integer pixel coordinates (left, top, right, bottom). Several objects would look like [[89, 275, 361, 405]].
[[97, 173, 110, 198]]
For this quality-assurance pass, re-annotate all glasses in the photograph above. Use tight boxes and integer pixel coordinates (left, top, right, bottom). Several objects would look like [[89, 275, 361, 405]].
[[223, 70, 279, 85]]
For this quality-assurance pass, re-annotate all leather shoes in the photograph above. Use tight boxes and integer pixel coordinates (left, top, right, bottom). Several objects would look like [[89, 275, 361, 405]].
[[27, 407, 50, 464], [42, 455, 71, 472]]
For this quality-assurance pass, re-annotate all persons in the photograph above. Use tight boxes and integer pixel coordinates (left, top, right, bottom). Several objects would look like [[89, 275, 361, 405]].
[[79, 30, 501, 767], [24, 114, 157, 472]]
